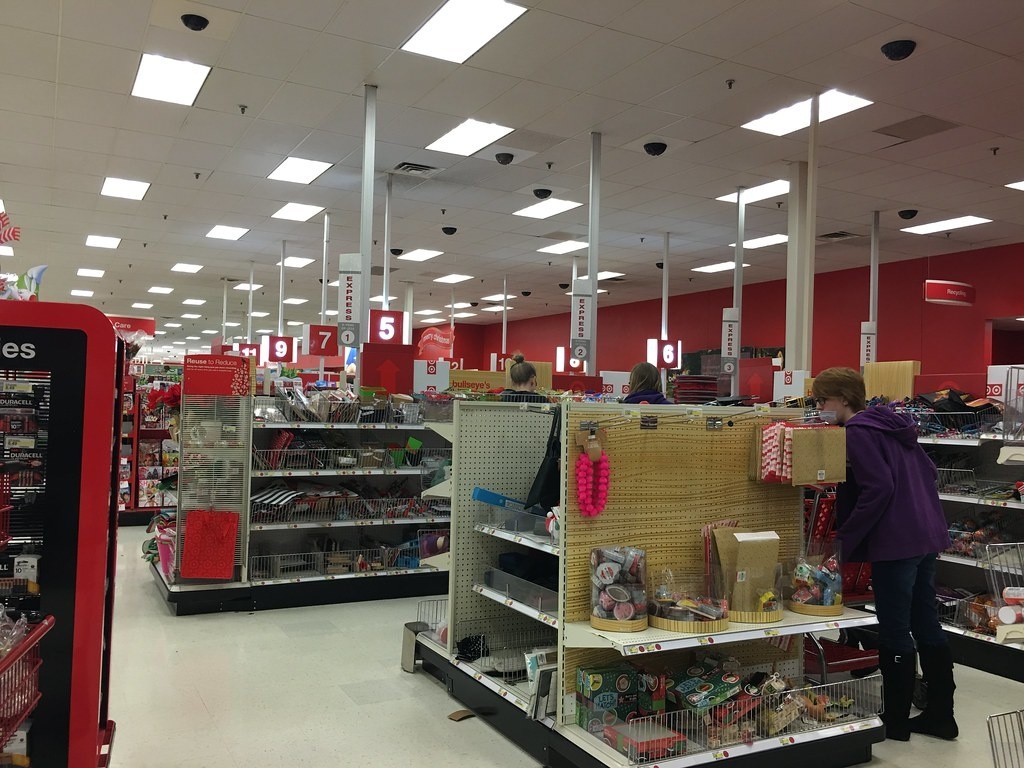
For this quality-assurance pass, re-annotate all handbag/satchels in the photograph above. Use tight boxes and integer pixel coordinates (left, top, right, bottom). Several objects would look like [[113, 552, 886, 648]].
[[523, 406, 561, 513]]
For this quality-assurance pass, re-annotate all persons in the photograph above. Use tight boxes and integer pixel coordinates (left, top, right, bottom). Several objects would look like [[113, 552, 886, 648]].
[[812, 367, 958, 740], [501, 354, 553, 403], [622, 362, 673, 404]]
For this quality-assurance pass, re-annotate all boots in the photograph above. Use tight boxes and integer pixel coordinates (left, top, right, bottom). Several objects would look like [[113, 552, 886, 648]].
[[908, 634, 958, 739], [877, 645, 916, 742]]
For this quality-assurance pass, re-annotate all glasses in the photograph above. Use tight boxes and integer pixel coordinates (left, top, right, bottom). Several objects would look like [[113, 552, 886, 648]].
[[814, 395, 844, 406]]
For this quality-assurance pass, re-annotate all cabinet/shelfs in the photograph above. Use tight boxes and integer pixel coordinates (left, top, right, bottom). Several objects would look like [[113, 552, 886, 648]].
[[0, 298, 1024, 768]]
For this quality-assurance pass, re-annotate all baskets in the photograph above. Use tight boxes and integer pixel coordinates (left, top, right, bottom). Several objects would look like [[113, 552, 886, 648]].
[[0, 614, 55, 751]]
